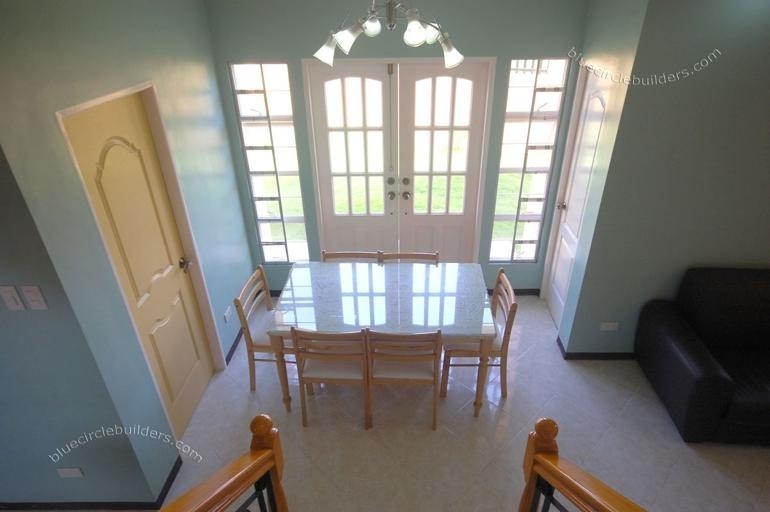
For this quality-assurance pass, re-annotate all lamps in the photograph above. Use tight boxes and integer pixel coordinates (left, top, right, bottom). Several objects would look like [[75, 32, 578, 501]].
[[310, 2, 466, 70]]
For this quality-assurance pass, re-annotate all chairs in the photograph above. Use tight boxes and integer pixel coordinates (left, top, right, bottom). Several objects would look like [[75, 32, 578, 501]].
[[230, 249, 517, 431], [633, 267, 770, 448]]
